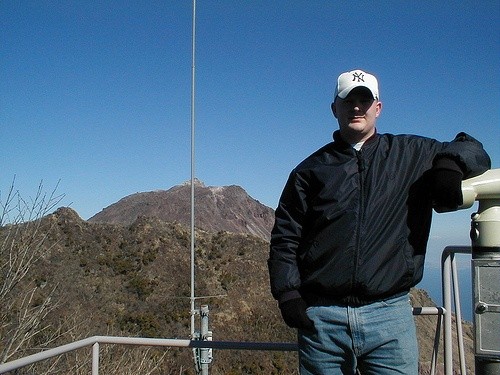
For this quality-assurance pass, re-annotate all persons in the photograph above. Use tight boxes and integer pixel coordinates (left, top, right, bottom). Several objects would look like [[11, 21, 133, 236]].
[[266, 69, 491, 375]]
